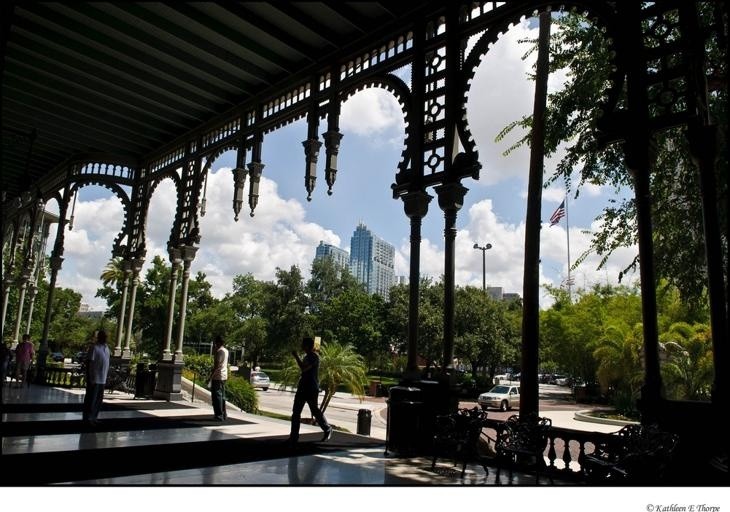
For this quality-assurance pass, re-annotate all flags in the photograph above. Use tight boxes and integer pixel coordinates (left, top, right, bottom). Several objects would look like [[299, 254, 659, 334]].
[[549, 199, 565, 226]]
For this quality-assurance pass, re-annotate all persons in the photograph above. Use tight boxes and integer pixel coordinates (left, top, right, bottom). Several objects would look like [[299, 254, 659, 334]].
[[289, 338, 331, 447], [0, 343, 13, 387], [83, 329, 109, 423], [13, 334, 34, 389], [205, 335, 229, 422]]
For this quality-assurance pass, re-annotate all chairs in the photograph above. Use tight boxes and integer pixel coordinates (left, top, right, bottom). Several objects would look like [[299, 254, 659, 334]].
[[430, 408, 680, 487]]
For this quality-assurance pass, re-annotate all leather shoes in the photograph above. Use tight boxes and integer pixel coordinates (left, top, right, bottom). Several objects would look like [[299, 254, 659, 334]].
[[321, 426, 333, 442]]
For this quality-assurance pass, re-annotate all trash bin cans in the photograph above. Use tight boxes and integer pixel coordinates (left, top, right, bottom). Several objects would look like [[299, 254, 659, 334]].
[[133, 369, 160, 400], [384, 386, 424, 458], [357, 409, 371, 435]]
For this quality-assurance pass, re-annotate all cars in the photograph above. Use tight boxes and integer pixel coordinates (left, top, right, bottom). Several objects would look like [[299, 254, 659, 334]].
[[494, 372, 575, 388], [478, 385, 520, 412], [70, 351, 88, 364], [50, 353, 64, 362], [250, 371, 270, 391]]
